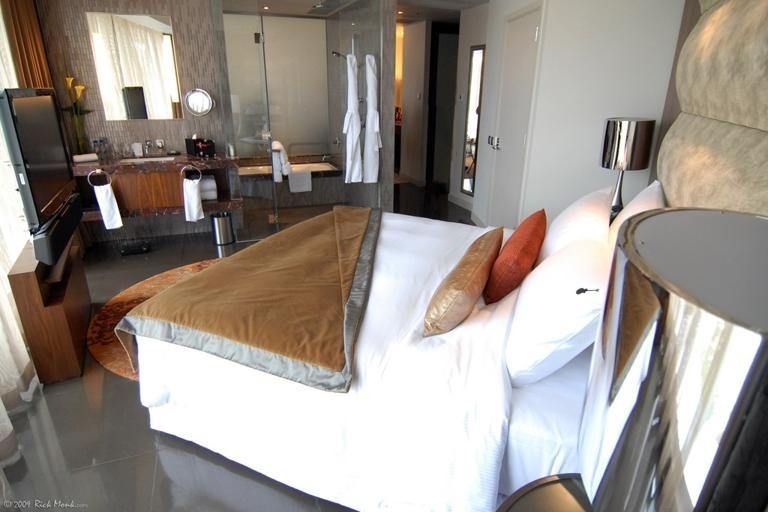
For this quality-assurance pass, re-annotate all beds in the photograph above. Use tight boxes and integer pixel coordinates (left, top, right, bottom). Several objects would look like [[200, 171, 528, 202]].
[[133, 203, 597, 512]]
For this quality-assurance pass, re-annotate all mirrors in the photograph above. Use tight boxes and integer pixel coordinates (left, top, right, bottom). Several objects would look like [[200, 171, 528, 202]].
[[460, 41, 486, 197], [183, 88, 214, 118], [84, 9, 186, 121]]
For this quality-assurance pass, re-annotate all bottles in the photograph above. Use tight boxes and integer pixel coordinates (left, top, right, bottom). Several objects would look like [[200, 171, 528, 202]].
[[94, 140, 108, 161]]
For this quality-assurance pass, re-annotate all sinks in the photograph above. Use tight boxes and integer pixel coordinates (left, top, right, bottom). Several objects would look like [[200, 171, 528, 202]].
[[120, 157, 175, 163]]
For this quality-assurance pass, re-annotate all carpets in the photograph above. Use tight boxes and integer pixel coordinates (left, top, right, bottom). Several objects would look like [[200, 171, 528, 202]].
[[84, 257, 230, 379]]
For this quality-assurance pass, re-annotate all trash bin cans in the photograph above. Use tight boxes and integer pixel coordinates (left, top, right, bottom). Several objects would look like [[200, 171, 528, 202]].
[[210, 212, 235, 246]]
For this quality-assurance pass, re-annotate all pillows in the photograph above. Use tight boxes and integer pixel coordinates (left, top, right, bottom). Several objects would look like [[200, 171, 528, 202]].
[[506, 178, 667, 391]]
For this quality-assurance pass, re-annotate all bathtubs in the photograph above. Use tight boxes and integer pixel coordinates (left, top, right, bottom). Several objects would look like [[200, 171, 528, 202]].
[[238, 162, 338, 175]]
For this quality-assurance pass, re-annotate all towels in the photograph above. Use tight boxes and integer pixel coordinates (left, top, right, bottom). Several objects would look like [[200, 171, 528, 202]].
[[72, 152, 99, 163], [271, 140, 290, 183], [182, 173, 219, 224], [287, 169, 313, 194], [92, 184, 124, 230]]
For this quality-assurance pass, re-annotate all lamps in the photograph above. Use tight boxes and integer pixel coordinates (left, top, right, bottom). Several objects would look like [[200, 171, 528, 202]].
[[600, 116, 658, 225], [574, 202, 767, 511]]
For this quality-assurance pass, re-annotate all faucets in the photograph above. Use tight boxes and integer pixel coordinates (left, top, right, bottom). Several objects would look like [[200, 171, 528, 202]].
[[145, 140, 152, 154], [322, 155, 331, 160]]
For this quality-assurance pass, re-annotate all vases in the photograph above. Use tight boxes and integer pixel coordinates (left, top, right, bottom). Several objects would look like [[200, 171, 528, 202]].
[[72, 115, 91, 154]]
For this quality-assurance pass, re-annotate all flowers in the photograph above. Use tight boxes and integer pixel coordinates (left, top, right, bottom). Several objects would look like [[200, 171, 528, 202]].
[[62, 75, 96, 153]]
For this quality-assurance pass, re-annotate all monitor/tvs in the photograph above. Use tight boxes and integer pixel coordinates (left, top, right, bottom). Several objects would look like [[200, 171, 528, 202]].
[[122, 86, 148, 119], [0, 87, 77, 237]]
